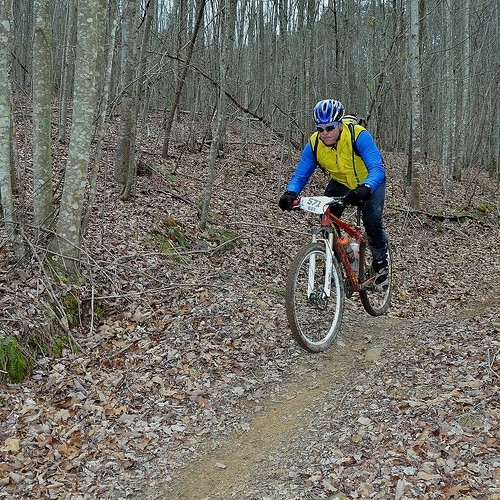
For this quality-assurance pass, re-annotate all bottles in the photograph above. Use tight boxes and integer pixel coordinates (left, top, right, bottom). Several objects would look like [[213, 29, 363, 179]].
[[340, 238, 355, 264]]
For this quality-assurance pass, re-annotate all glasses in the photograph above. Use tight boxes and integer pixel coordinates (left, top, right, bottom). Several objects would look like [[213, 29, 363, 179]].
[[316, 125, 336, 132]]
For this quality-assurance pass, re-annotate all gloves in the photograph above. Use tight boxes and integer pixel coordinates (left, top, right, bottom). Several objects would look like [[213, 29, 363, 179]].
[[343, 185, 369, 205], [279, 192, 296, 212]]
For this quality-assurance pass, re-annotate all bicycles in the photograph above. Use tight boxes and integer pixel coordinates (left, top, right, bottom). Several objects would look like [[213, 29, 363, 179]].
[[276, 194, 394, 353]]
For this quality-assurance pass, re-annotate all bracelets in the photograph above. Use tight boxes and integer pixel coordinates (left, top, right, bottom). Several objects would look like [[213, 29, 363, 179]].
[[363, 183, 373, 191]]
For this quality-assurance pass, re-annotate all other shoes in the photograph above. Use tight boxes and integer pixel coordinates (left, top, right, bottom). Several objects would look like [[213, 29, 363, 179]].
[[373, 266, 389, 291]]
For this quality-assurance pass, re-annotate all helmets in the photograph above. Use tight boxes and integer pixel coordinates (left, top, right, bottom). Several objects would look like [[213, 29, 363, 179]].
[[313, 98, 345, 124]]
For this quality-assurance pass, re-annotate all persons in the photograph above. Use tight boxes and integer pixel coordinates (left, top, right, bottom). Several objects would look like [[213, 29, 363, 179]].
[[278, 99, 390, 293]]
